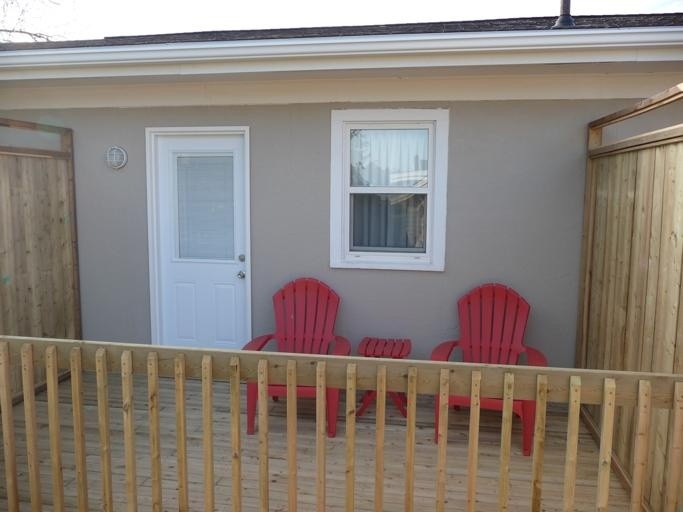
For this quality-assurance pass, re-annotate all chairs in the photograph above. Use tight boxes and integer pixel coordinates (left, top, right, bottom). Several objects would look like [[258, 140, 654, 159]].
[[431, 283, 547, 457], [241, 278, 352, 438]]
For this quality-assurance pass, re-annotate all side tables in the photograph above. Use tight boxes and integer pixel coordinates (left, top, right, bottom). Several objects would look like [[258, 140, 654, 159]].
[[356, 337, 412, 417]]
